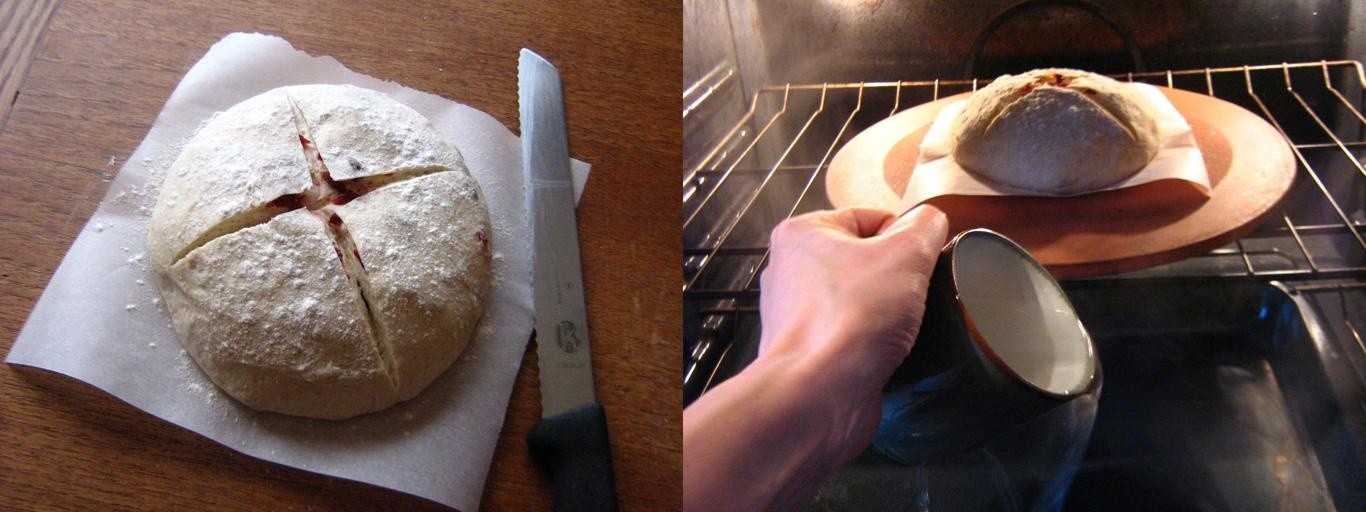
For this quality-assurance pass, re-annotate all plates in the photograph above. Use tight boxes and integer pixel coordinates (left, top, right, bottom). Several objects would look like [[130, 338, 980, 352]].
[[826, 83, 1301, 275]]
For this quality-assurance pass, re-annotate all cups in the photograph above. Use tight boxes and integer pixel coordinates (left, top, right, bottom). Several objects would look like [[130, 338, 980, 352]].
[[866, 227, 1098, 464]]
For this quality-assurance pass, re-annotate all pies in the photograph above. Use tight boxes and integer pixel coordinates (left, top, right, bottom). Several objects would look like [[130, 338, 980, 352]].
[[153, 85, 491, 418], [954, 68, 1159, 193]]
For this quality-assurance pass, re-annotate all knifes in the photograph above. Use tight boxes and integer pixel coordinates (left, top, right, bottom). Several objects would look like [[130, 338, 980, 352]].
[[515, 46, 620, 510]]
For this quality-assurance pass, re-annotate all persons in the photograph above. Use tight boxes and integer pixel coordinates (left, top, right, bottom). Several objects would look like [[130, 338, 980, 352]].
[[682, 205, 950, 511]]
